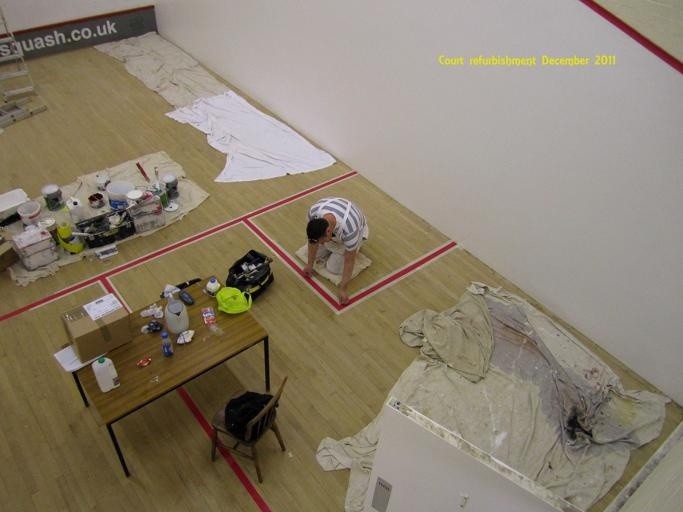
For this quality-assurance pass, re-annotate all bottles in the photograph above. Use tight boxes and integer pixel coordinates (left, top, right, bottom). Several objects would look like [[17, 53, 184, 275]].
[[206, 278, 220, 298]]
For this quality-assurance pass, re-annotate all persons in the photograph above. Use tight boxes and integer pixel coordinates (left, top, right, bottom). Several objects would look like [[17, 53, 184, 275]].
[[303, 197, 368, 305]]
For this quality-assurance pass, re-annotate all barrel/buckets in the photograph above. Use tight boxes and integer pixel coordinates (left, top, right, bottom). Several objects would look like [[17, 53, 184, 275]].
[[65, 197, 84, 223], [91, 357, 121, 392], [16, 200, 41, 226], [107, 181, 134, 209], [41, 184, 65, 212]]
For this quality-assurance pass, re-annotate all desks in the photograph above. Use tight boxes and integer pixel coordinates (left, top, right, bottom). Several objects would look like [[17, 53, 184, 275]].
[[62, 275, 270, 477]]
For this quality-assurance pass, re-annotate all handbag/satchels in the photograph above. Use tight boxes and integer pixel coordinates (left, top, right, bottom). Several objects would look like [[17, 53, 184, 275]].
[[226, 250, 274, 303], [225, 392, 278, 440]]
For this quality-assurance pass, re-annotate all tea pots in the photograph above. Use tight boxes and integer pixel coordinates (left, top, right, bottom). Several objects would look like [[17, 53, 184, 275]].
[[163, 292, 189, 336]]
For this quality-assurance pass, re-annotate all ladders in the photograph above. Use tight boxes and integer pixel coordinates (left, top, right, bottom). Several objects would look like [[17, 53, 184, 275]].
[[0, 0, 47, 129]]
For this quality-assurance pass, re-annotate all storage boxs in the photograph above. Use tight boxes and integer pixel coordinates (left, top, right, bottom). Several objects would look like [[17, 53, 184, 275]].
[[0, 188, 61, 270], [60, 292, 133, 362]]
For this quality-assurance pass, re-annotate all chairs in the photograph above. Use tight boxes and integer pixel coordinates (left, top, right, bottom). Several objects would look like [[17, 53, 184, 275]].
[[209, 373, 288, 482]]
